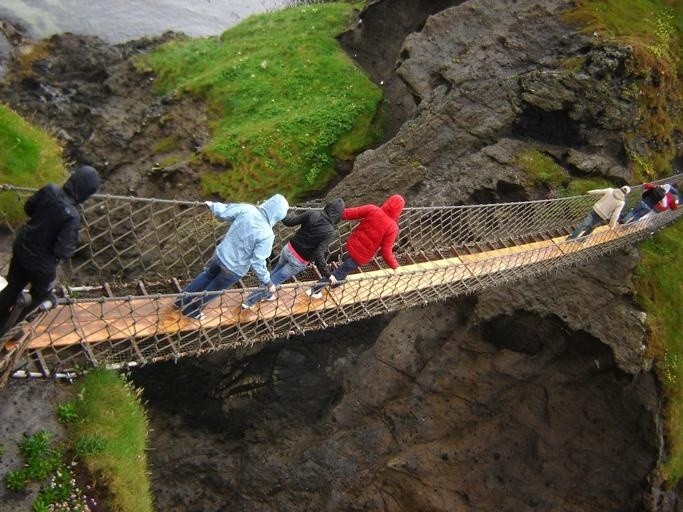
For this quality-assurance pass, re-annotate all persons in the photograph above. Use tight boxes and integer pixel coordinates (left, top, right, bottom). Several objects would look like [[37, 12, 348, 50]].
[[175, 193, 289, 319], [619, 180, 680, 230], [305, 194, 405, 299], [0, 166, 100, 323], [242, 198, 346, 310], [565, 185, 630, 244]]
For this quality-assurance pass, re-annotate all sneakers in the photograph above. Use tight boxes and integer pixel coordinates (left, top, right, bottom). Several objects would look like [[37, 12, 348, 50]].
[[242, 303, 256, 309], [196, 311, 206, 321], [305, 288, 323, 299], [266, 293, 276, 302]]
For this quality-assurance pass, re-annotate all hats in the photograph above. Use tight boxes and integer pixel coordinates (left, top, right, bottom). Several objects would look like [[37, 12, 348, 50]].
[[621, 185, 631, 194]]
